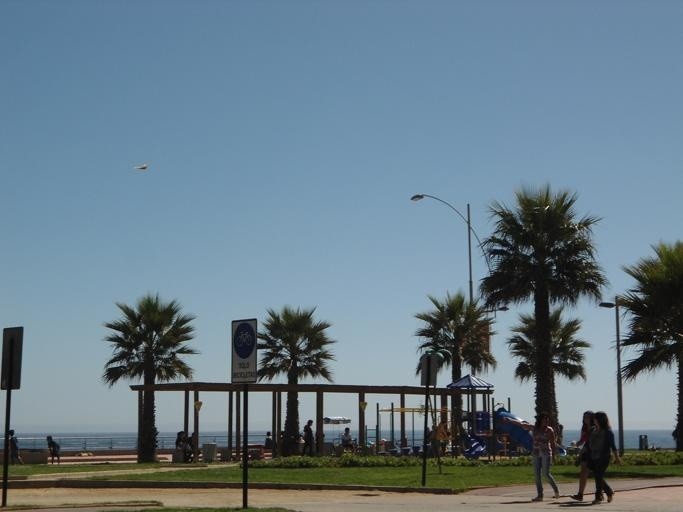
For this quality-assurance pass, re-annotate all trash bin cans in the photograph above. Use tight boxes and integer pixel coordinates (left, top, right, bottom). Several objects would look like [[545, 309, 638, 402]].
[[639, 435, 649, 450]]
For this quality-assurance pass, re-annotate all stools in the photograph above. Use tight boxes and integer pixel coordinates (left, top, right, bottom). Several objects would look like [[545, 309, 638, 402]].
[[264, 448, 272, 460]]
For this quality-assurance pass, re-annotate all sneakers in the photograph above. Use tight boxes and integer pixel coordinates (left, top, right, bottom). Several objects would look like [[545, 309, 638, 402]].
[[532, 497, 543, 501], [554, 488, 559, 499], [608, 491, 615, 502], [592, 500, 602, 504], [570, 494, 583, 501]]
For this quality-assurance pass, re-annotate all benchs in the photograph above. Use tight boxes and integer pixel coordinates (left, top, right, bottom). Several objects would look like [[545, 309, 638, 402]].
[[334, 438, 365, 456], [172, 446, 206, 463], [216, 447, 244, 461]]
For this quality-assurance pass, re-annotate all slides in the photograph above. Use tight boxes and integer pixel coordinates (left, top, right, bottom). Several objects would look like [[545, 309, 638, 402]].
[[496, 410, 567, 457], [463, 442, 488, 458]]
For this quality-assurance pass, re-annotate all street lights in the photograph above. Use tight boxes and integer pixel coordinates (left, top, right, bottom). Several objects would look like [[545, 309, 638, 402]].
[[599, 296, 625, 456], [409, 194, 509, 314]]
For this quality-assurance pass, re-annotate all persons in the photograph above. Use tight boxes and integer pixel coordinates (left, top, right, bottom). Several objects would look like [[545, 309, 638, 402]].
[[46, 436, 60, 465], [264, 419, 314, 459], [342, 428, 354, 453], [532, 413, 560, 502], [429, 425, 440, 458], [426, 427, 430, 444], [570, 410, 594, 502], [175, 431, 201, 464], [586, 411, 622, 504], [8, 429, 24, 465]]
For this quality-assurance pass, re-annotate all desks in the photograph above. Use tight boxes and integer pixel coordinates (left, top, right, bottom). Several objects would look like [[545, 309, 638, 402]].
[[248, 444, 265, 460]]
